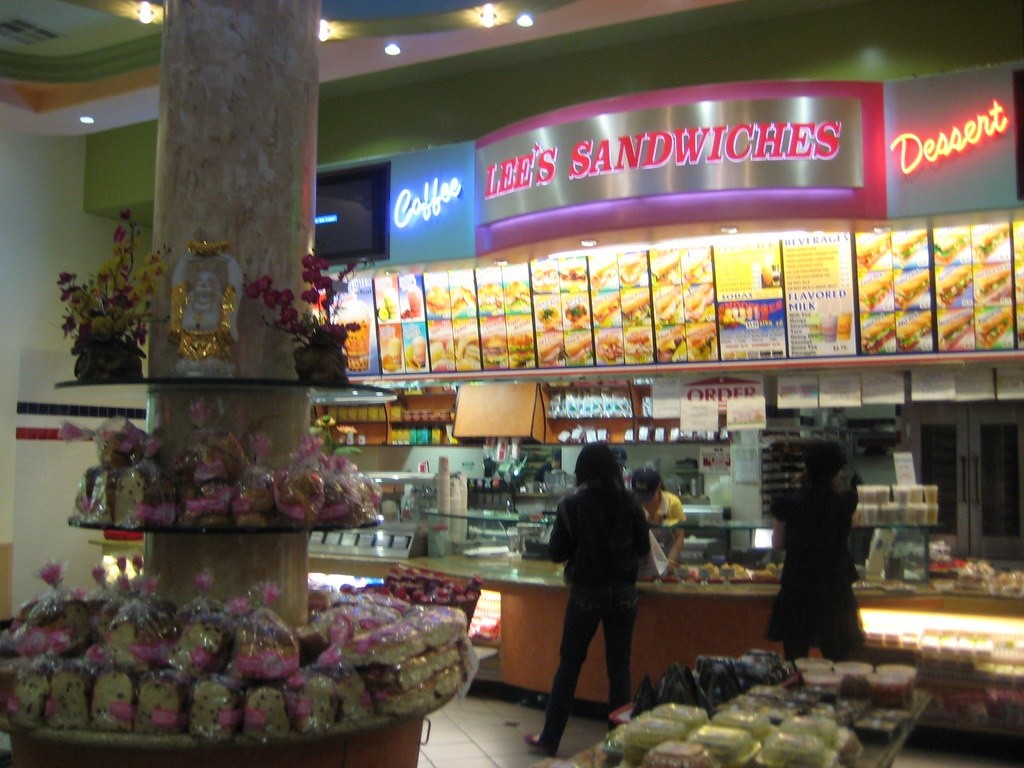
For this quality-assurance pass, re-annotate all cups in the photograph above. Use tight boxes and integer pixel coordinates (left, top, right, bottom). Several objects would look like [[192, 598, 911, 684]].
[[853, 484, 939, 524], [436, 455, 467, 511]]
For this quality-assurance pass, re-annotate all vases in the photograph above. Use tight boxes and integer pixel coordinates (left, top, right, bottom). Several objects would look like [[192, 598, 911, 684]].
[[73, 344, 141, 380], [285, 345, 356, 383]]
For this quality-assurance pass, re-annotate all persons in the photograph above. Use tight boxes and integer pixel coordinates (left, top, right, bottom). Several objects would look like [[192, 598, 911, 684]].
[[533, 447, 687, 578], [765, 438, 867, 664], [523, 441, 651, 756]]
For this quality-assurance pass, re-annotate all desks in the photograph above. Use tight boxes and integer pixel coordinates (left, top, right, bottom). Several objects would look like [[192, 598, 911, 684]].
[[560, 674, 933, 768]]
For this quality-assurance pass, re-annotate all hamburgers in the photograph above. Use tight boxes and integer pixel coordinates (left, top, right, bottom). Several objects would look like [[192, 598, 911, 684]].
[[427, 246, 533, 369]]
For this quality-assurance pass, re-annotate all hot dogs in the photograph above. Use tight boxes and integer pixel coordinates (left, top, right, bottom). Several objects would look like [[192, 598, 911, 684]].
[[856, 224, 1024, 353], [531, 249, 716, 364]]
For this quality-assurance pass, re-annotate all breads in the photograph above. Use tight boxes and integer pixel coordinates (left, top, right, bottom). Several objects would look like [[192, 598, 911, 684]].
[[0, 594, 472, 741], [75, 428, 380, 529]]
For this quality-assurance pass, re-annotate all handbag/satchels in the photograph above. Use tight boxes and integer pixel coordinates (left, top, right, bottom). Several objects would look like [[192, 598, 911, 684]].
[[638, 529, 669, 581]]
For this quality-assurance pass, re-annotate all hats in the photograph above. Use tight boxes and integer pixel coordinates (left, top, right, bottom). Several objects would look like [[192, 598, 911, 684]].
[[632, 467, 660, 504]]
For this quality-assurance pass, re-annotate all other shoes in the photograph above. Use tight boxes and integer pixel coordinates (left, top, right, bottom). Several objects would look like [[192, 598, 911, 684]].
[[524, 733, 541, 748]]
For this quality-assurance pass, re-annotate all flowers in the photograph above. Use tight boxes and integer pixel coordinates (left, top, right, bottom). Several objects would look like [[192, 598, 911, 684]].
[[57, 207, 171, 358], [246, 249, 361, 345]]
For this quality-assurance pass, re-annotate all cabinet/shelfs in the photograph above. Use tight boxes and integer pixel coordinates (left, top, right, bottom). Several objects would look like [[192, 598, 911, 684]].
[[319, 394, 456, 446], [732, 428, 858, 554], [542, 378, 730, 444], [857, 578, 1024, 761]]
